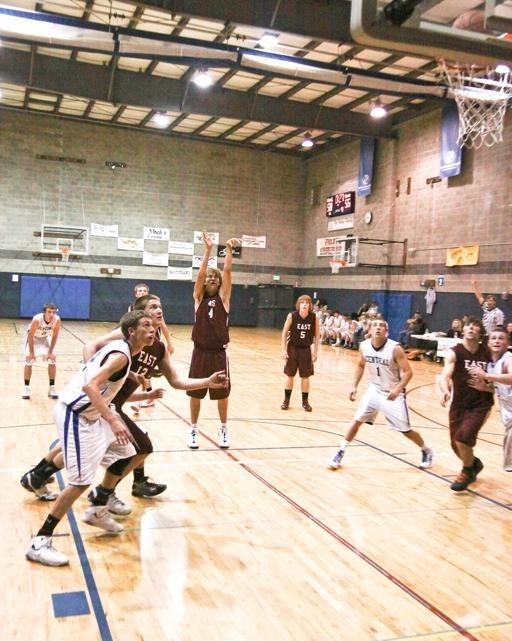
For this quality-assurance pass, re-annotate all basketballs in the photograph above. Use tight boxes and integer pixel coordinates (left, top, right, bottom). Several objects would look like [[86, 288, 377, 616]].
[[454, 10, 499, 37]]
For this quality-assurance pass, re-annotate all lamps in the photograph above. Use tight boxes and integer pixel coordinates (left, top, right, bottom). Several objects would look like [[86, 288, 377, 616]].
[[301, 133, 313, 148], [370, 97, 387, 119]]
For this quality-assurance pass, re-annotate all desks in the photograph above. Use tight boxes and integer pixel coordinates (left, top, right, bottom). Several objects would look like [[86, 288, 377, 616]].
[[409, 335, 460, 350]]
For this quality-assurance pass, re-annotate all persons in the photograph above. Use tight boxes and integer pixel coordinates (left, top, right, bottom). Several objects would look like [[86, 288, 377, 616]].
[[438, 318, 493, 492], [186, 229, 245, 451], [126, 282, 174, 415], [308, 272, 512, 365], [21, 303, 65, 401], [465, 325, 512, 471], [329, 320, 432, 471], [278, 295, 319, 411], [21, 294, 231, 502], [28, 309, 156, 568]]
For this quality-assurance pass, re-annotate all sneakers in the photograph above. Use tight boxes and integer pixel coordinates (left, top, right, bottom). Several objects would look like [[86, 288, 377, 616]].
[[328, 448, 344, 470], [301, 401, 312, 412], [280, 398, 290, 409], [218, 425, 230, 448], [129, 400, 140, 413], [19, 467, 168, 568], [450, 457, 484, 493], [21, 385, 31, 399], [319, 338, 359, 351], [418, 447, 435, 469], [47, 385, 58, 399], [186, 424, 201, 448], [139, 396, 155, 409]]
